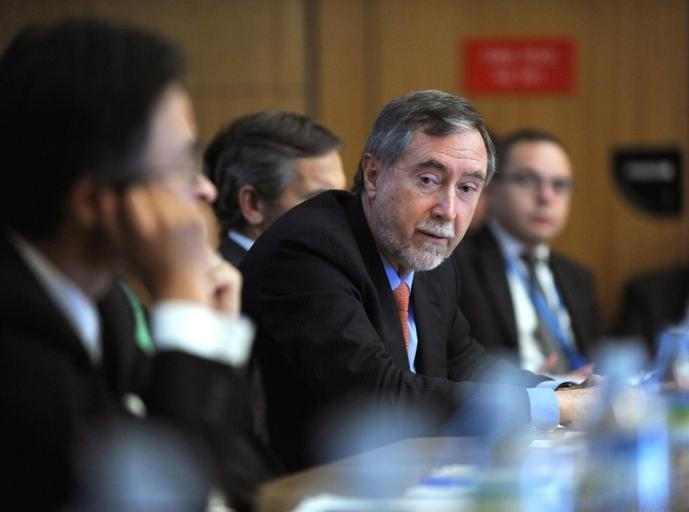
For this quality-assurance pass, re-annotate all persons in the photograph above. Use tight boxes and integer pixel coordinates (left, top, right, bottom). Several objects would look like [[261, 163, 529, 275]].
[[234, 89, 679, 479], [614, 260, 689, 365], [0, 17, 268, 512], [202, 111, 348, 269], [451, 126, 604, 378]]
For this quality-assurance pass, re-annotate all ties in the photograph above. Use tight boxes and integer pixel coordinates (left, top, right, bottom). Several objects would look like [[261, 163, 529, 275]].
[[394, 282, 410, 350], [522, 254, 587, 369]]
